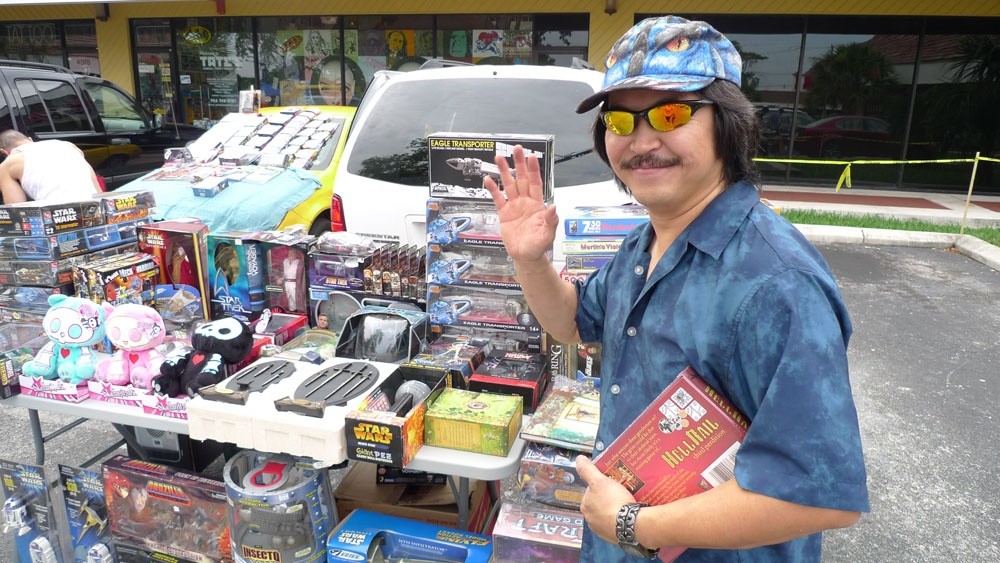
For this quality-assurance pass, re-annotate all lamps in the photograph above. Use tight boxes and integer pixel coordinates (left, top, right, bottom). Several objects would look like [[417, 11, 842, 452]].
[[94, 3, 111, 26], [604, 1, 620, 18]]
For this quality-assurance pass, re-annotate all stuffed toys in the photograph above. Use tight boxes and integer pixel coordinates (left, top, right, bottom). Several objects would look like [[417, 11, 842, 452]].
[[21, 293, 253, 400]]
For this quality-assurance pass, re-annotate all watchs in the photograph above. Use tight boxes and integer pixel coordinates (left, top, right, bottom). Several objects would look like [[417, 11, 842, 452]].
[[615, 501, 661, 561]]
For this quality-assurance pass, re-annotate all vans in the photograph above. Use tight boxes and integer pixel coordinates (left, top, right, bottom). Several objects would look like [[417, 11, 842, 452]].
[[329, 65, 647, 267]]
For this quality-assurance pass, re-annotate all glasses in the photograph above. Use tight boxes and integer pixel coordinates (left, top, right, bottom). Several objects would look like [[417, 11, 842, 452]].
[[596, 98, 718, 137]]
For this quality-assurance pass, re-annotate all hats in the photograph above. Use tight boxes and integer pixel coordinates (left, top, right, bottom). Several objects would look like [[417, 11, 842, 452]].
[[574, 15, 743, 115]]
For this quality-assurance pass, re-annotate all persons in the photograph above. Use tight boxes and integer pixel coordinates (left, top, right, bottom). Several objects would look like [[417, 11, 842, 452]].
[[1, 129, 104, 205], [312, 313, 330, 331], [483, 12, 869, 562], [277, 247, 303, 312]]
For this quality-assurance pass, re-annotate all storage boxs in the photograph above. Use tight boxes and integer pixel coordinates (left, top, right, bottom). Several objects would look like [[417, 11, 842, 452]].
[[0, 129, 597, 562]]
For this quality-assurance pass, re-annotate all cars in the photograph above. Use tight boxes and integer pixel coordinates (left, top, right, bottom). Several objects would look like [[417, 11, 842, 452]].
[[108, 106, 358, 236], [795, 115, 940, 159]]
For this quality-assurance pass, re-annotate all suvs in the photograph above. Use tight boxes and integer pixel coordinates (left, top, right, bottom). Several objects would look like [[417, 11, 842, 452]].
[[753, 103, 817, 150], [0, 58, 206, 193]]
